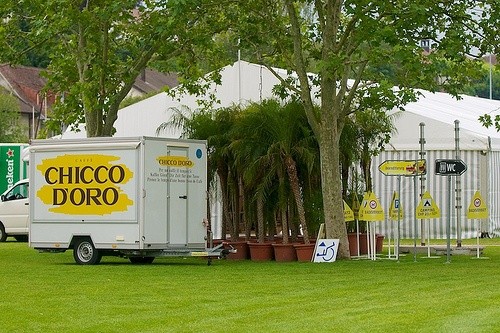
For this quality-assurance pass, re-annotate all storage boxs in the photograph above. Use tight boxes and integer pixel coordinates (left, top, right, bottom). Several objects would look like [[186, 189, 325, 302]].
[[27, 136, 209, 252]]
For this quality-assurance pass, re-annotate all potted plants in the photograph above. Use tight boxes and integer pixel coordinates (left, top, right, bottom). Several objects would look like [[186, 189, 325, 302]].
[[176, 100, 387, 263]]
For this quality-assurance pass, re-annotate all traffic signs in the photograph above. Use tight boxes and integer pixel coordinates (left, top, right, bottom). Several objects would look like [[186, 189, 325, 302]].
[[434, 159, 467, 176], [378, 160, 427, 176]]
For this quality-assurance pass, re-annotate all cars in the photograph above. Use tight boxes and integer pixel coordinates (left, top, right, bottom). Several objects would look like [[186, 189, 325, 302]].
[[0, 179, 29, 243]]
